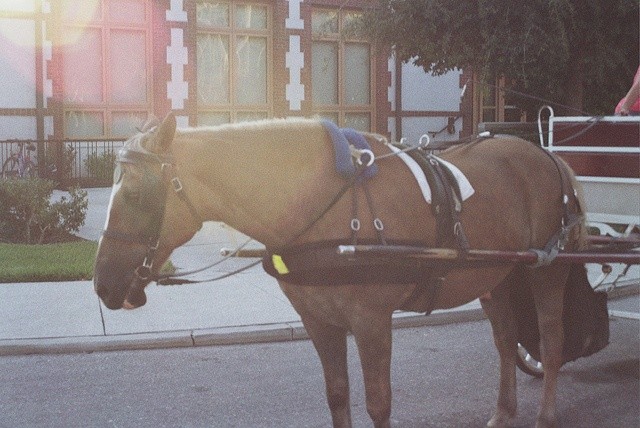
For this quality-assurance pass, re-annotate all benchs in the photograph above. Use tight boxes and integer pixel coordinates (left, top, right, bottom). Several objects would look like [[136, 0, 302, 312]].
[[537, 104, 639, 237]]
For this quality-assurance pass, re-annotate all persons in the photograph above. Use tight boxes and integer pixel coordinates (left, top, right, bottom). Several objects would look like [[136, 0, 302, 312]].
[[615, 64, 640, 115]]
